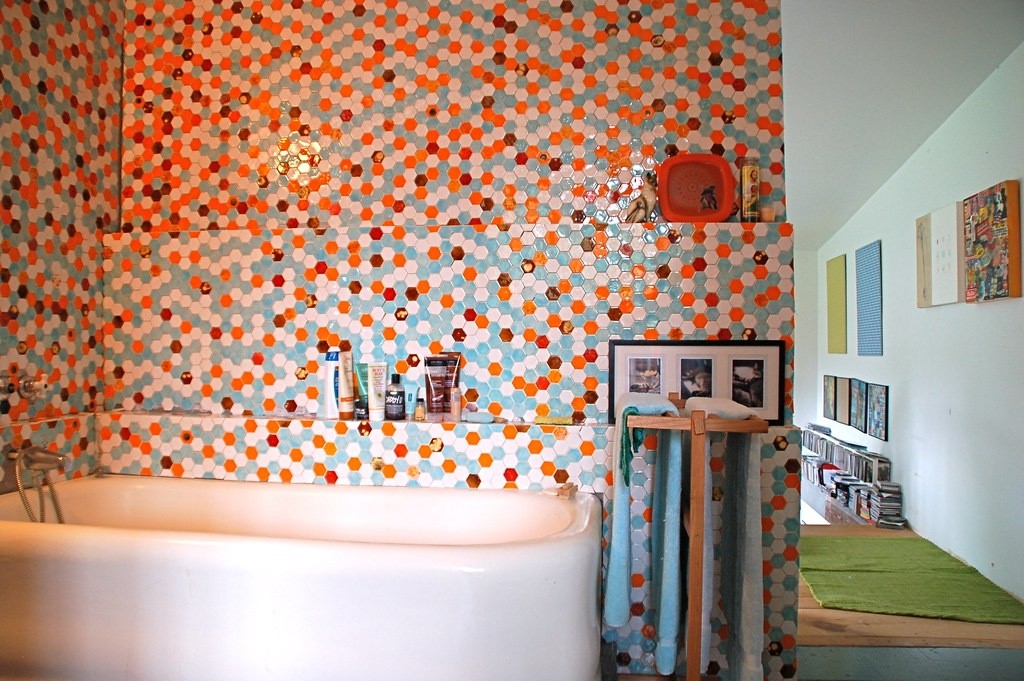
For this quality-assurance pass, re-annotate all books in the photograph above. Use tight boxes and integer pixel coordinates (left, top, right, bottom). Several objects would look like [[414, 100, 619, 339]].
[[801, 423, 907, 529]]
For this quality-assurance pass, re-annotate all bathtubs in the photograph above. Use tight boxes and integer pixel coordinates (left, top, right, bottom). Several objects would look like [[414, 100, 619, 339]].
[[0, 470, 602, 681]]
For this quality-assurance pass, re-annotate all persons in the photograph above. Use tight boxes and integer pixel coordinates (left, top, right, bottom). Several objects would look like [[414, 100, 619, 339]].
[[732, 361, 763, 407]]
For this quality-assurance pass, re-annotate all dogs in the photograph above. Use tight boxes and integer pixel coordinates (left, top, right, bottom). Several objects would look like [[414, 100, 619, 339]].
[[625, 172, 658, 223]]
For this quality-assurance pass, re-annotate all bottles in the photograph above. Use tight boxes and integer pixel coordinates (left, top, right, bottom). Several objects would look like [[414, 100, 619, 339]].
[[386, 374, 403, 420], [415, 399, 425, 421]]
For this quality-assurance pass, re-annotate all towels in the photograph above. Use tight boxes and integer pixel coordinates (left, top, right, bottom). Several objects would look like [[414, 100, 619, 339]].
[[602, 391, 683, 675], [683, 396, 764, 681]]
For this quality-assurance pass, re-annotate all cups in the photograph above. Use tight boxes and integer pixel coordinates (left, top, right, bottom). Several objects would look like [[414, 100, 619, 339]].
[[760, 203, 776, 222], [739, 157, 761, 223]]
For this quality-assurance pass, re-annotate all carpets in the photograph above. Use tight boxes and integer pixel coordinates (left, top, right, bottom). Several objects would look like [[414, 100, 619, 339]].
[[794, 536, 1024, 624]]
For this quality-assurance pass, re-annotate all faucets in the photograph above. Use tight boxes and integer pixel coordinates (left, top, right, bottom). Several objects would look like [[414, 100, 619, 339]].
[[5, 444, 72, 474]]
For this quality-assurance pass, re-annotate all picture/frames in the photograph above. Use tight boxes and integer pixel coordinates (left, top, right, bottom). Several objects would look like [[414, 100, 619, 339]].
[[849, 377, 868, 434], [866, 383, 890, 442], [823, 375, 837, 421], [608, 338, 785, 427], [835, 377, 851, 426]]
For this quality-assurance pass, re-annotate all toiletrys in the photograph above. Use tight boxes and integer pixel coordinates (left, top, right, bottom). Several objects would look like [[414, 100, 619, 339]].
[[323, 349, 463, 422]]
